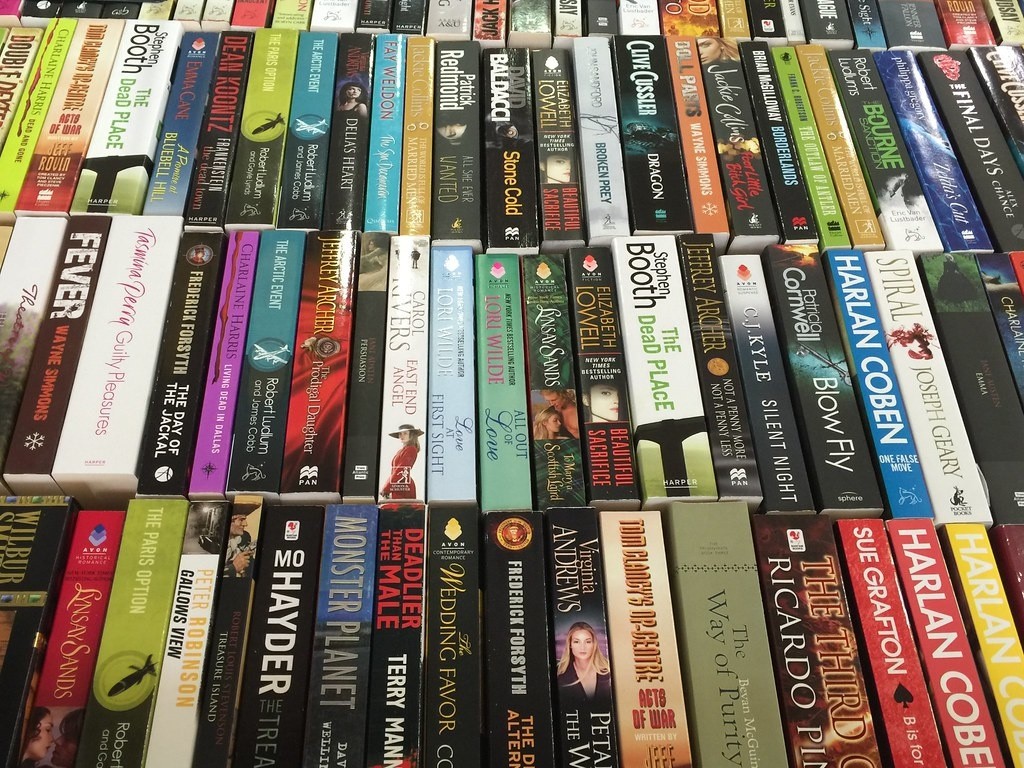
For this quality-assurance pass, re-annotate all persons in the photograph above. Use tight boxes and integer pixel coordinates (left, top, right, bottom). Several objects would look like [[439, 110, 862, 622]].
[[51, 708, 83, 767], [19, 706, 53, 767], [539, 389, 580, 438], [379, 424, 423, 499], [538, 148, 574, 183], [698, 37, 741, 65], [435, 113, 469, 145], [583, 381, 621, 424], [358, 238, 387, 291], [338, 82, 368, 116], [411, 246, 420, 269], [533, 406, 570, 438], [936, 254, 978, 303], [556, 622, 611, 707], [223, 504, 255, 577]]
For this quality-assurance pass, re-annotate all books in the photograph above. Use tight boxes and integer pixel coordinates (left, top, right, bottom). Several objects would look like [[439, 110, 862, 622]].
[[0, 0, 1023, 767]]
